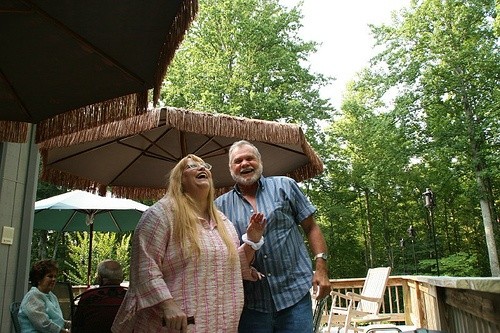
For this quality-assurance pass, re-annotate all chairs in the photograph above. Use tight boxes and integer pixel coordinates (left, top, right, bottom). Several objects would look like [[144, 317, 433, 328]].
[[9, 302, 21, 333], [324, 266, 392, 333], [28, 282, 75, 321]]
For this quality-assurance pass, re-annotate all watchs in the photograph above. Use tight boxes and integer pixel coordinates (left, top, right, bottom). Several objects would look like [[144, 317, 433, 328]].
[[314, 253, 327, 262]]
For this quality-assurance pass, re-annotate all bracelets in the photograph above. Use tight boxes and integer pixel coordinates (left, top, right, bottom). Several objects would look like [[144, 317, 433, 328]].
[[242, 233, 264, 251]]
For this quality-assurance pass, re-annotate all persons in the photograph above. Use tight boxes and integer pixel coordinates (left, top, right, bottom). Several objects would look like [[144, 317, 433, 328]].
[[72, 261, 127, 333], [214, 140, 331, 333], [18, 258, 72, 333], [112, 155, 267, 333]]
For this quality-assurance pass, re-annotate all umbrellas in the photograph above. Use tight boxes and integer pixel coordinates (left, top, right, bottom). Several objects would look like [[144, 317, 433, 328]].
[[40, 107, 322, 200], [34, 190, 150, 288]]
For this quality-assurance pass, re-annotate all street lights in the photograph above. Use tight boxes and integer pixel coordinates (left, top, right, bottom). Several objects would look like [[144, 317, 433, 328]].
[[423, 187, 439, 276], [407, 224, 419, 275], [399, 237, 406, 274]]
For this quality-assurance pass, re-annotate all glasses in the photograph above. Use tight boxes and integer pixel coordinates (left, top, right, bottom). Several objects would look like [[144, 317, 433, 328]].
[[43, 274, 57, 279], [182, 162, 212, 170]]
